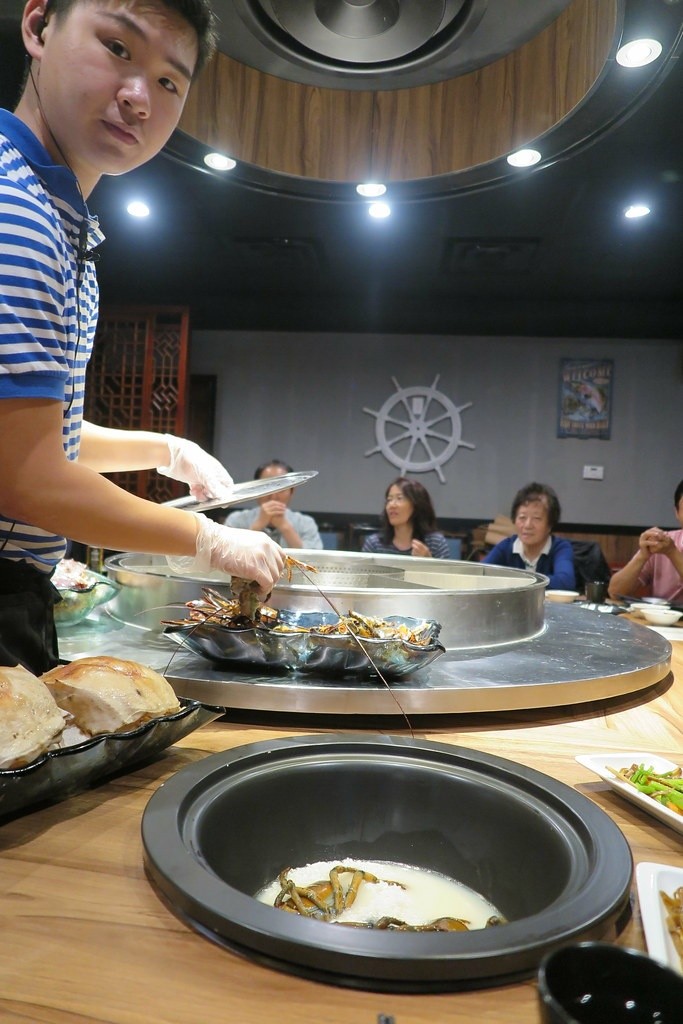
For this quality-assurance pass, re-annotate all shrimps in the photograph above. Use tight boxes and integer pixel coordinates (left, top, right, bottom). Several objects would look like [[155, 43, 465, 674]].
[[163, 588, 419, 660], [230, 550, 318, 626]]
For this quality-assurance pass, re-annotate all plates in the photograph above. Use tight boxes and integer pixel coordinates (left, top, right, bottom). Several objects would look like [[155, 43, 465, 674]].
[[635, 862, 683, 978], [573, 751, 683, 837]]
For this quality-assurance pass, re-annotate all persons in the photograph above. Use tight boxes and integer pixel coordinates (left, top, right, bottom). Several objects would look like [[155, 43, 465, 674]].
[[606, 480, 683, 604], [361, 478, 449, 560], [0, 0, 286, 677], [481, 483, 576, 590], [224, 460, 322, 551]]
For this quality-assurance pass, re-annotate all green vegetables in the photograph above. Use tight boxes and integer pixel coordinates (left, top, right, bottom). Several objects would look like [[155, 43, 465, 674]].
[[629, 763, 683, 819]]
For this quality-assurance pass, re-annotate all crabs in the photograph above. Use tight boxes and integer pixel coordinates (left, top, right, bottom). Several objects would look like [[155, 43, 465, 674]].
[[340, 912, 507, 933], [274, 865, 403, 924]]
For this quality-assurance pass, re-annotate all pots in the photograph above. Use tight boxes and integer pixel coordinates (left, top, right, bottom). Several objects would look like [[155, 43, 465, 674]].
[[102, 547, 550, 648], [141, 732, 635, 993]]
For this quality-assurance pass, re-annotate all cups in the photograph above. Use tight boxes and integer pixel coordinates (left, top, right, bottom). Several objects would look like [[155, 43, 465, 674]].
[[584, 580, 608, 604]]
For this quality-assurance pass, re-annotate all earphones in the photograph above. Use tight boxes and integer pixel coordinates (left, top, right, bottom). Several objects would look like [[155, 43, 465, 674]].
[[36, 20, 48, 44]]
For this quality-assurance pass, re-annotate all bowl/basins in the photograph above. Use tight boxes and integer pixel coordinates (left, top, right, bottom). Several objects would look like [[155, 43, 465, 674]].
[[631, 603, 671, 617], [162, 608, 447, 679], [640, 609, 683, 627], [546, 589, 579, 603], [0, 693, 226, 828], [534, 939, 683, 1024], [53, 569, 123, 628]]
